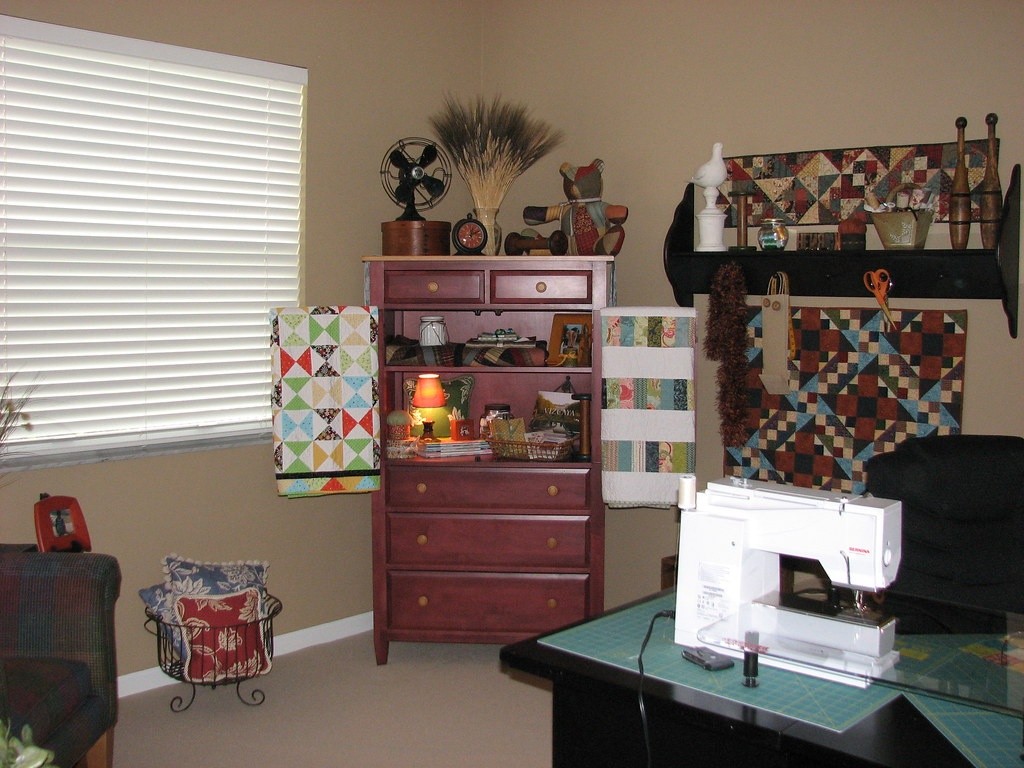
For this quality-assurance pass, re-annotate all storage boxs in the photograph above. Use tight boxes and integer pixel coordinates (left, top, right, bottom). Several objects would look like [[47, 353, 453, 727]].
[[870, 211, 934, 248]]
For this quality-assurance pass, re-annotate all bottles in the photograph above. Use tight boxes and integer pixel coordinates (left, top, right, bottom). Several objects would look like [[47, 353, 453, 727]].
[[480, 405, 516, 440], [420, 317, 448, 346], [759, 218, 789, 251]]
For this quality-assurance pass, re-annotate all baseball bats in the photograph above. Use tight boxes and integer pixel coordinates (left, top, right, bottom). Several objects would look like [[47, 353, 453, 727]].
[[948, 113, 1003, 250]]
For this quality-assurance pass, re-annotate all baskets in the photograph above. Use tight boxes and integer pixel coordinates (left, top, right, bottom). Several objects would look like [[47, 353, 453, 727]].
[[485, 436, 577, 462]]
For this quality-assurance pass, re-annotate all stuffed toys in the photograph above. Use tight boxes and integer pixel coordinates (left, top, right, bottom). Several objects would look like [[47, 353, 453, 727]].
[[522, 157, 629, 257]]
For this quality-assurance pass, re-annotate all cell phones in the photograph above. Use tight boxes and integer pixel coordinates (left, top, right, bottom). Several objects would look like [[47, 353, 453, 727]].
[[681, 646, 735, 671]]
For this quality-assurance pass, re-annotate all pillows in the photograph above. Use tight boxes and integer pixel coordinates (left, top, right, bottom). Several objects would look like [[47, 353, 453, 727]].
[[160, 553, 271, 642], [174, 587, 273, 682], [403, 375, 475, 437], [138, 582, 188, 660]]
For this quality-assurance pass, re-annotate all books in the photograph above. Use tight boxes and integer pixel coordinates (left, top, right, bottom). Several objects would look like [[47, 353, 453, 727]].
[[464, 337, 537, 349], [416, 435, 493, 458]]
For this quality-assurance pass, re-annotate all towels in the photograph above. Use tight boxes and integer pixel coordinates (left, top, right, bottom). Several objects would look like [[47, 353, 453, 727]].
[[599, 306, 699, 509], [268, 305, 382, 499]]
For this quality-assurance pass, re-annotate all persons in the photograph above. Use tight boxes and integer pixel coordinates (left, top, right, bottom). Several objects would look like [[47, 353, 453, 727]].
[[53, 511, 67, 535], [561, 325, 580, 353]]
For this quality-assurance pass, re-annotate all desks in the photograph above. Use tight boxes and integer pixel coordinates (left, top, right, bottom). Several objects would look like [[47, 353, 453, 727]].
[[501, 570, 1024, 768]]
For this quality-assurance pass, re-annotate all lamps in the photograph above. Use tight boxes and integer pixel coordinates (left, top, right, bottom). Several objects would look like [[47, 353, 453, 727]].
[[411, 373, 446, 442]]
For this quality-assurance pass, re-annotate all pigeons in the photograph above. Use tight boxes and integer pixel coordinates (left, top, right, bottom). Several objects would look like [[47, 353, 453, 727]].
[[684, 143, 728, 188]]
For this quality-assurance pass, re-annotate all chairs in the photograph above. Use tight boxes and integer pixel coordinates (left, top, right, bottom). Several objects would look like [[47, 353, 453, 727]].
[[0, 552, 122, 768]]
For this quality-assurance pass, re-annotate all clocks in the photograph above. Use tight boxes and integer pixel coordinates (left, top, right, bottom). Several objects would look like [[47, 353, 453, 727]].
[[452, 213, 488, 255]]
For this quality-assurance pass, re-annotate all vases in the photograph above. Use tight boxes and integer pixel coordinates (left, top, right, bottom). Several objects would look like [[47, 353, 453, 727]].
[[474, 206, 502, 255]]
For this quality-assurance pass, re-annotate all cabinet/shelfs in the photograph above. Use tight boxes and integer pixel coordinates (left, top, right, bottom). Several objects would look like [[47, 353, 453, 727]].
[[362, 254, 616, 665]]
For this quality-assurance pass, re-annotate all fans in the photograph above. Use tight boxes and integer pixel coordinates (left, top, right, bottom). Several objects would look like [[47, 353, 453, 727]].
[[379, 137, 453, 221]]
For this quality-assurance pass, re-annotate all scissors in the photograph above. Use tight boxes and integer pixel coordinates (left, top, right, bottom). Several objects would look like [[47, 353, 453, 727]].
[[862, 269, 897, 330]]
